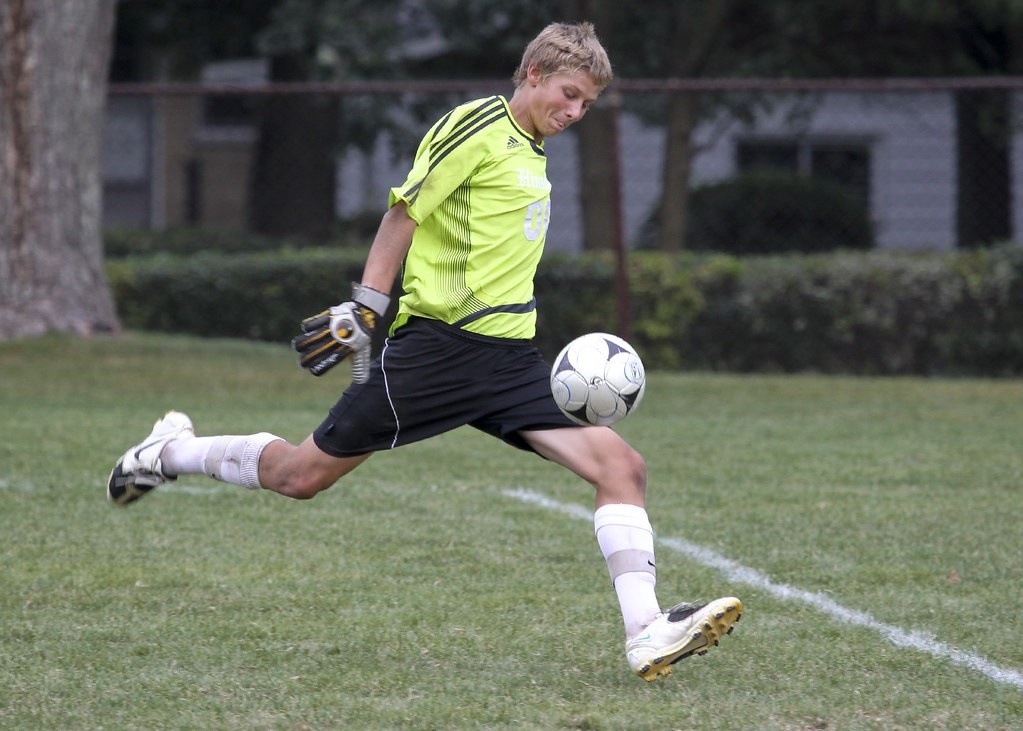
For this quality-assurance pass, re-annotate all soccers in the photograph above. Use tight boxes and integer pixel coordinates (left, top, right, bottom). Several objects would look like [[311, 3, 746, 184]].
[[550, 330, 651, 424]]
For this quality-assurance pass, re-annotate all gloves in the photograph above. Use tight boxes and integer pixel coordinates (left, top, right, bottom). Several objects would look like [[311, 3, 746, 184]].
[[291, 282, 391, 387]]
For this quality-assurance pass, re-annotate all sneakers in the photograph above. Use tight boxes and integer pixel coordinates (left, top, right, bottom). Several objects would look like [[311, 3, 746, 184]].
[[106, 409, 199, 506], [624, 594, 743, 683]]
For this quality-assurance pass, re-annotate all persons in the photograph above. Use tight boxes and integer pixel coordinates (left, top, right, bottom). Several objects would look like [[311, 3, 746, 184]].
[[107, 23, 743, 682]]
